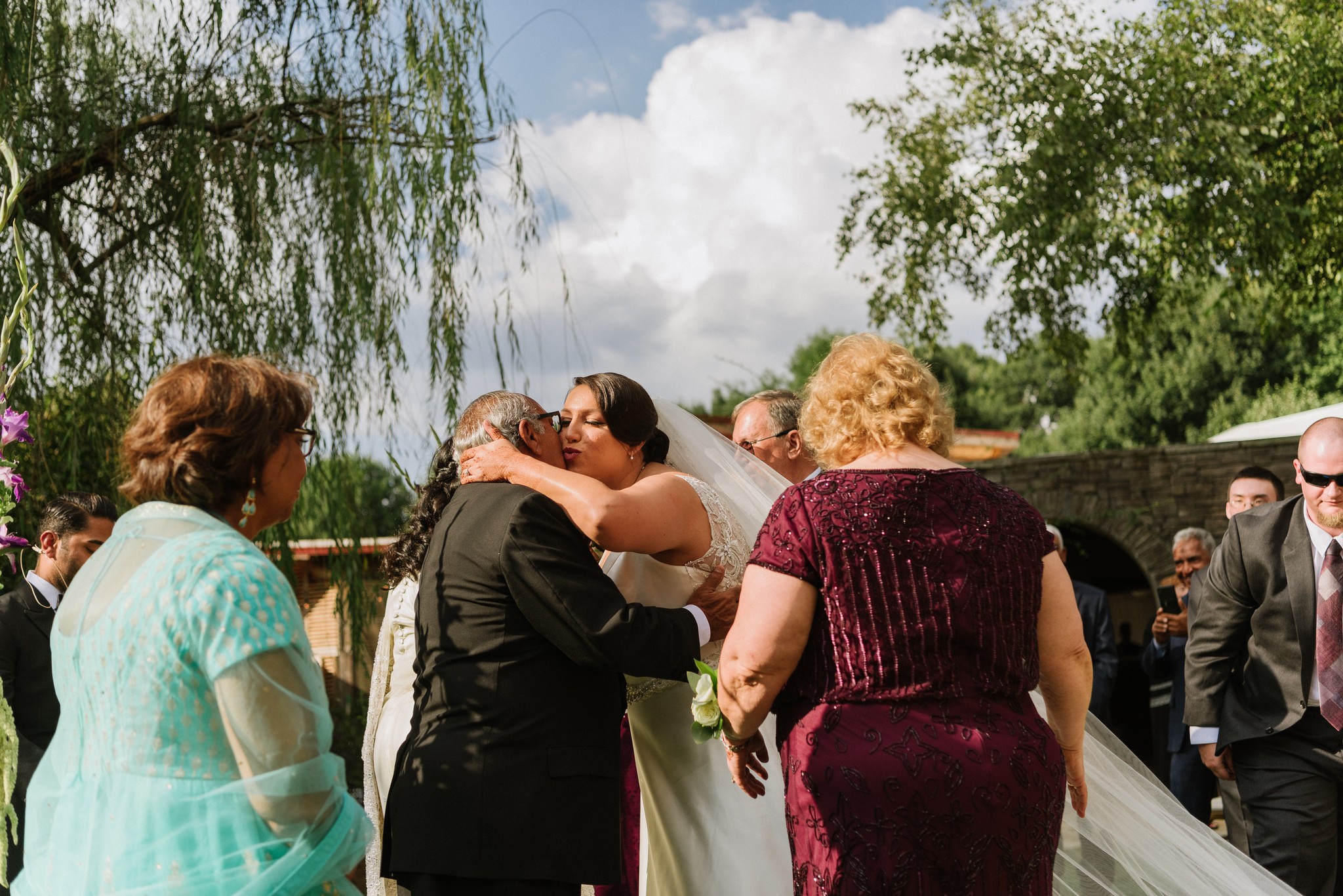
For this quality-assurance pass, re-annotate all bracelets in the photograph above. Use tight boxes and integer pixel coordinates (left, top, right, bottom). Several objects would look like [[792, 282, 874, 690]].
[[719, 730, 760, 752]]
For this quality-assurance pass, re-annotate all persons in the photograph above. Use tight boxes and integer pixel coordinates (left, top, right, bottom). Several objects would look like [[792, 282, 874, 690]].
[[347, 391, 745, 896], [446, 362, 803, 896], [1181, 413, 1343, 896], [691, 331, 1097, 896], [1173, 464, 1289, 849], [1125, 525, 1232, 840], [1034, 508, 1159, 786], [0, 490, 124, 896], [293, 411, 472, 896], [6, 351, 377, 896], [722, 386, 825, 487]]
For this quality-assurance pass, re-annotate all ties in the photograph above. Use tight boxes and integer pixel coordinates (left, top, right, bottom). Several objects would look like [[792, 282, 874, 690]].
[[1315, 540, 1343, 732]]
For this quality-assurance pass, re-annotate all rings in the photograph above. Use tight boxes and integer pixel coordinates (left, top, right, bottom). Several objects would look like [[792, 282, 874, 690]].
[[1073, 786, 1077, 790], [464, 467, 470, 477], [1067, 781, 1074, 787], [730, 776, 737, 784]]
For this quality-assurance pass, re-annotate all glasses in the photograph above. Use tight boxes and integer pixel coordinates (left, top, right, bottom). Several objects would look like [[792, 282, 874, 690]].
[[532, 410, 564, 435], [737, 427, 798, 456], [287, 426, 317, 458], [1298, 461, 1343, 489]]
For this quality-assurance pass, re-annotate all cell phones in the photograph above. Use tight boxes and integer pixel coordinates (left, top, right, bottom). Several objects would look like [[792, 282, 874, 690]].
[[1158, 584, 1181, 617]]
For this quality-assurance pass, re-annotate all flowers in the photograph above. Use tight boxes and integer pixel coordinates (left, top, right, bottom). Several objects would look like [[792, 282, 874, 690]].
[[689, 662, 758, 750]]
